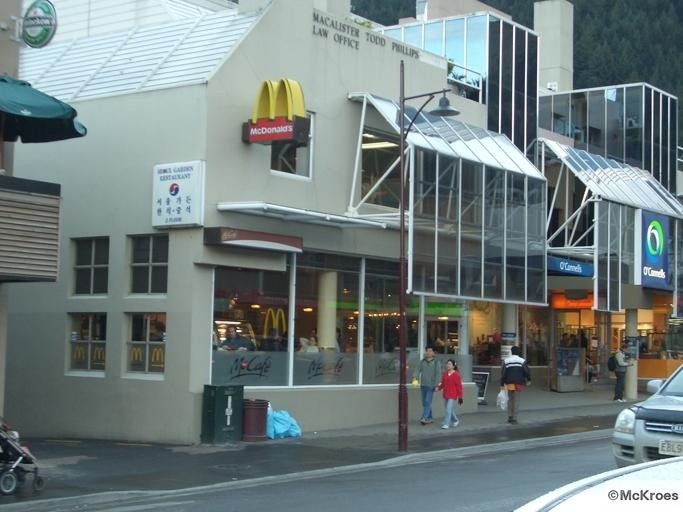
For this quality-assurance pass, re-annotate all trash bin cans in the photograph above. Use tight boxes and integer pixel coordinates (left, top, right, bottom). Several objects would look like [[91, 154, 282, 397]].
[[204, 384, 244, 442]]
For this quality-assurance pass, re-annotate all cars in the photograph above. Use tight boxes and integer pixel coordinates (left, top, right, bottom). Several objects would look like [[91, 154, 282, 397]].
[[514, 364, 683, 512]]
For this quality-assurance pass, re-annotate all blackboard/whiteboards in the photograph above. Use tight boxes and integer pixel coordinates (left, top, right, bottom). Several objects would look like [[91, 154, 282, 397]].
[[472, 372, 489, 400]]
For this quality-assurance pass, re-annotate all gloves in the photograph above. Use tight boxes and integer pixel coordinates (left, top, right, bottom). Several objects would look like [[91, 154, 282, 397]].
[[457, 398, 463, 406]]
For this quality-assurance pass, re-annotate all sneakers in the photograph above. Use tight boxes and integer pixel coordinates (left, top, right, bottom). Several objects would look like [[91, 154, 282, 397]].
[[418, 417, 460, 430], [613, 398, 627, 403]]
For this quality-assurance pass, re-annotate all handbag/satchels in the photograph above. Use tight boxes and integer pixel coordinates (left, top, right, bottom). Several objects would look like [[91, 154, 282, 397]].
[[496, 387, 508, 410]]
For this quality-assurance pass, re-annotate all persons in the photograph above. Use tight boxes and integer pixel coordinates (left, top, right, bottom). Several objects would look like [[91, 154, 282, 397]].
[[409, 344, 441, 425], [564, 333, 579, 376], [559, 332, 570, 373], [613, 342, 633, 403], [434, 357, 464, 430], [211, 320, 225, 352], [218, 325, 255, 351], [259, 327, 457, 360], [499, 346, 532, 425], [577, 327, 587, 349]]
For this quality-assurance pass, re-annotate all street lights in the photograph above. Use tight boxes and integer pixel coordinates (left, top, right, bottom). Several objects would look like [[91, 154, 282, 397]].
[[398, 58, 462, 459]]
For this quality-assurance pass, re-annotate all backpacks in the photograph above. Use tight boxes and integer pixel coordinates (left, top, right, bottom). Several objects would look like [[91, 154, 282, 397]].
[[607, 351, 620, 371]]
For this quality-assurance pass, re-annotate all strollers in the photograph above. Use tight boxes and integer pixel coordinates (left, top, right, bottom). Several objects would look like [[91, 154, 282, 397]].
[[0, 417, 45, 495]]
